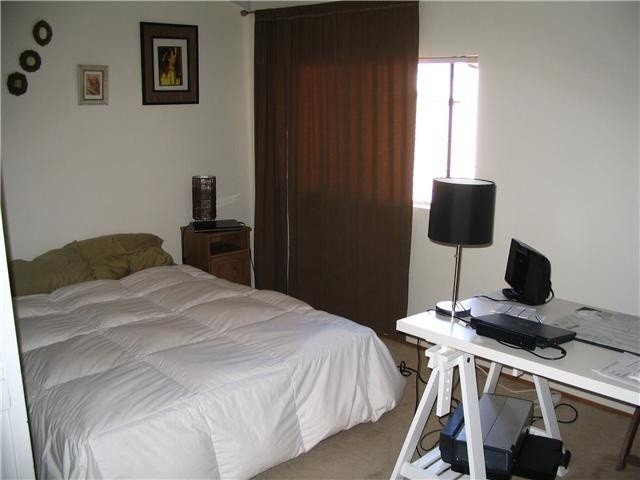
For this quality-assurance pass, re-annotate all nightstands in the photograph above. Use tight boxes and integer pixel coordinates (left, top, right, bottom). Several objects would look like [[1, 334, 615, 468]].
[[181, 222, 252, 288]]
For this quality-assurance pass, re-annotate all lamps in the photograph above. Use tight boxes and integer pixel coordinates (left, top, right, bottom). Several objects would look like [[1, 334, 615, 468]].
[[191, 173, 217, 229], [427, 177, 495, 315]]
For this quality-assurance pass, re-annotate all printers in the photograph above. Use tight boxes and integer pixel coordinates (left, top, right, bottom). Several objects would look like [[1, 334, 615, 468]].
[[439, 393, 570, 480]]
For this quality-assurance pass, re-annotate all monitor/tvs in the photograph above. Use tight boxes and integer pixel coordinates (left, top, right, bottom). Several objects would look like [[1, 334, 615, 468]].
[[504, 239, 551, 305]]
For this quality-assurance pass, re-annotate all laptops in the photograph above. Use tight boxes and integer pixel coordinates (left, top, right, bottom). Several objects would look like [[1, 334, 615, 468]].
[[471, 312, 577, 352], [189, 219, 242, 233]]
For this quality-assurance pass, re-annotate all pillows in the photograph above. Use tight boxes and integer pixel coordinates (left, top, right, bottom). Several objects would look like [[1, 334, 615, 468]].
[[10, 234, 176, 296]]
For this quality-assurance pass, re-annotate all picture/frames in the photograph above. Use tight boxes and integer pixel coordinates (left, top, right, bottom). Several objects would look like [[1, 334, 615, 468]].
[[140, 21, 200, 106], [77, 63, 109, 106]]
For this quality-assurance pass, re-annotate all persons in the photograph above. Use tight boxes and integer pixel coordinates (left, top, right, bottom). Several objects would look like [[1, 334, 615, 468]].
[[161, 48, 177, 85]]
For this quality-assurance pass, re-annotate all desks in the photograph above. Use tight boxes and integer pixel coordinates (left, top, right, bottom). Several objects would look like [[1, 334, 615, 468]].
[[391, 292, 639, 479]]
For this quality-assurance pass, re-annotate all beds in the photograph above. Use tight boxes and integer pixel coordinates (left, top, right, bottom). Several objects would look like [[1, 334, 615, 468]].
[[8, 234, 389, 479]]
